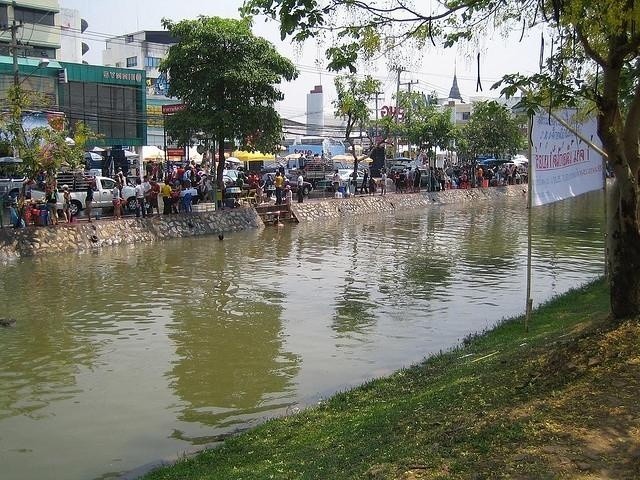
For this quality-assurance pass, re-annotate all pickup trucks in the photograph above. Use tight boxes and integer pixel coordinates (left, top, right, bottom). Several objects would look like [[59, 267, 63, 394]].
[[57, 176, 136, 217]]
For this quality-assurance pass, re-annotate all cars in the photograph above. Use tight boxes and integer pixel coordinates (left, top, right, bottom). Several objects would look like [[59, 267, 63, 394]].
[[262, 172, 312, 197], [348, 171, 377, 188]]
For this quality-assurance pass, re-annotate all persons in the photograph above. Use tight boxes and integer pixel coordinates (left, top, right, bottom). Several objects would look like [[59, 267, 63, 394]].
[[44, 153, 307, 225], [360, 164, 528, 196], [333, 169, 340, 192]]
